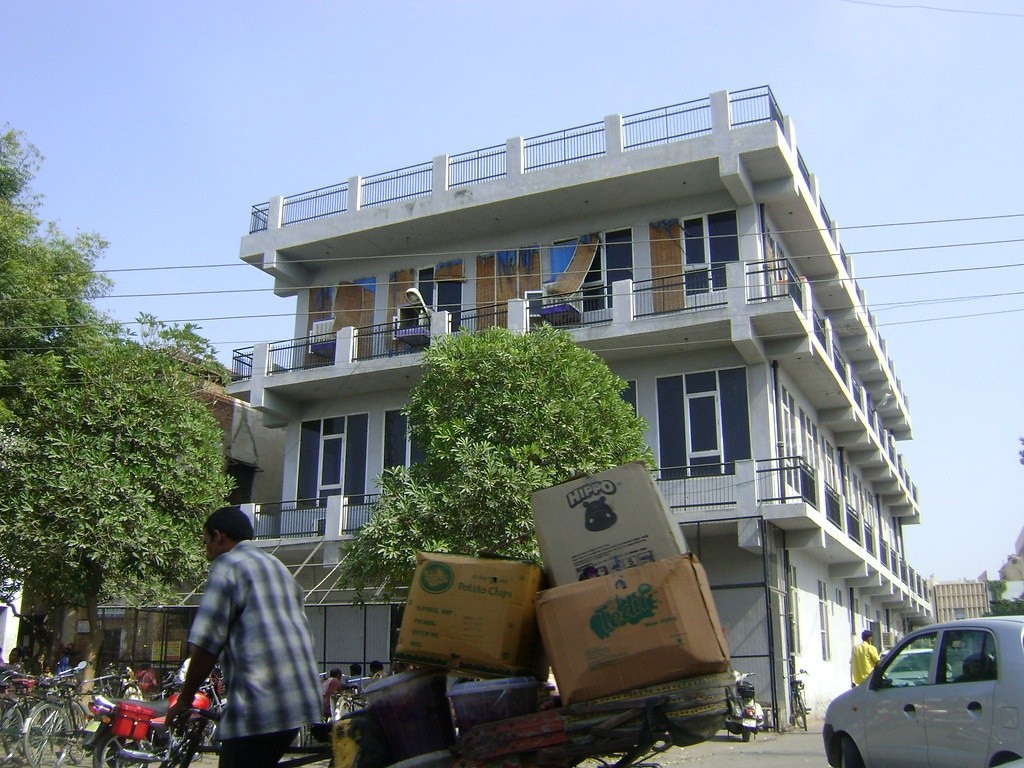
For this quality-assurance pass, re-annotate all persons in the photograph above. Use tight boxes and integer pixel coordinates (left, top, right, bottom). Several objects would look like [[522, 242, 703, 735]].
[[163, 507, 323, 768], [849, 630, 880, 690], [136, 663, 158, 697], [54, 647, 79, 688], [0, 646, 25, 693], [321, 660, 384, 723]]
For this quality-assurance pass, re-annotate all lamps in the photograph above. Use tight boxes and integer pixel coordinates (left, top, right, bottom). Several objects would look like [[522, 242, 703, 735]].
[[405, 288, 431, 323]]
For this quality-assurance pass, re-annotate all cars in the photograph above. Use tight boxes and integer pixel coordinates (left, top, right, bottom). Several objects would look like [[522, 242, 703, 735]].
[[876, 647, 956, 687], [823, 615, 1024, 768]]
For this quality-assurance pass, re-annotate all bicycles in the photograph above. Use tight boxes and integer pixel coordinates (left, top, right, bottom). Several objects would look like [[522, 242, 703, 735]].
[[781, 668, 811, 733], [0, 650, 384, 768]]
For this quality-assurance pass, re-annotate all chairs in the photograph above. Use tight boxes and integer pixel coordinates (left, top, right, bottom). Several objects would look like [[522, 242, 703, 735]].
[[953, 653, 994, 681]]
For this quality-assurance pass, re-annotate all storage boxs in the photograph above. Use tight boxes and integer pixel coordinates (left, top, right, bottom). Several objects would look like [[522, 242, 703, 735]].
[[533, 552, 731, 706], [393, 549, 554, 681], [530, 460, 689, 586]]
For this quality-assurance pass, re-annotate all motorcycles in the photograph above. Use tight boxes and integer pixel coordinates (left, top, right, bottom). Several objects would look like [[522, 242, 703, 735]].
[[726, 670, 765, 744], [85, 658, 237, 768]]
